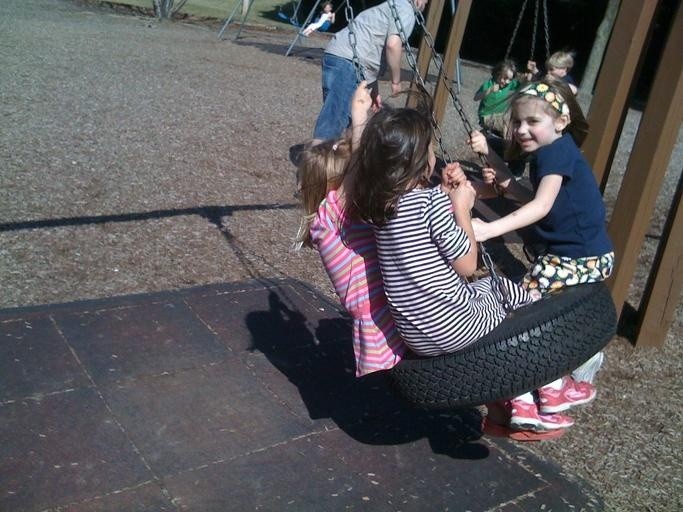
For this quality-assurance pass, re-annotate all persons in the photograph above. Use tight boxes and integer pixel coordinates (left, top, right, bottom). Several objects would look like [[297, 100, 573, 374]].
[[312, 0, 430, 147], [298, 2, 336, 37], [471, 75, 615, 384], [338, 89, 598, 433], [288, 136, 489, 442], [473, 60, 533, 180], [526, 49, 580, 96]]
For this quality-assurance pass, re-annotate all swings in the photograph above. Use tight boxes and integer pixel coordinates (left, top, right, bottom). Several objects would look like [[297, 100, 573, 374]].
[[345, 1, 616, 413], [315, 0, 346, 32], [479, 1, 550, 167]]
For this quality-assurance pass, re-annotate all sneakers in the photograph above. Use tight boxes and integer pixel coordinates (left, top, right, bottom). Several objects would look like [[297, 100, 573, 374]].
[[571, 351, 605, 383], [538, 376, 596, 414], [504, 395, 575, 432]]
